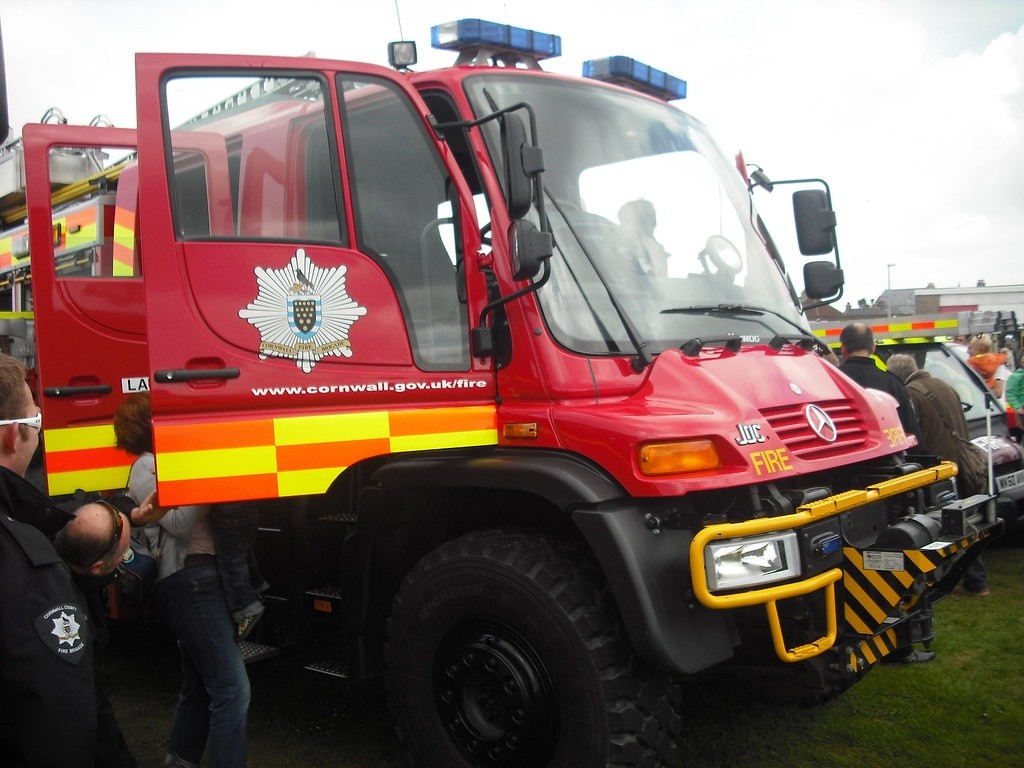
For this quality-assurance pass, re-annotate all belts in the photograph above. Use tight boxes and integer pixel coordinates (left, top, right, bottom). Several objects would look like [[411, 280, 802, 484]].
[[184, 557, 217, 567]]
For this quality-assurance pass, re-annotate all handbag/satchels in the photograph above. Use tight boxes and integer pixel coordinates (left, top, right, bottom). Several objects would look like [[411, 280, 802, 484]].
[[949, 430, 988, 497]]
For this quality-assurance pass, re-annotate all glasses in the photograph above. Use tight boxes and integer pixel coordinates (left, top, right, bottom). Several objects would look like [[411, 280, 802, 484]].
[[87, 499, 123, 568], [0, 412, 42, 433]]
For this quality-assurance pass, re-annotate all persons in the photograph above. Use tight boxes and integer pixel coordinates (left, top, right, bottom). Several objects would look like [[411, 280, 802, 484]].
[[0, 346, 284, 768], [838, 323, 1024, 596]]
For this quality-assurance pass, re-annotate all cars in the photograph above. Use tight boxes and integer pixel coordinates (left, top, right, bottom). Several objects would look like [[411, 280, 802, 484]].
[[820, 334, 1024, 546]]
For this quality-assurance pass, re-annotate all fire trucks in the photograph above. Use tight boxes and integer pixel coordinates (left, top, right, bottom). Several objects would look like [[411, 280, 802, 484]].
[[0, 19, 1002, 767]]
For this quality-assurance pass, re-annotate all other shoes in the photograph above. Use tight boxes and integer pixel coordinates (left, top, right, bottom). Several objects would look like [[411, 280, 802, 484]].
[[165, 751, 199, 768], [238, 611, 263, 642], [878, 649, 935, 666], [953, 584, 990, 597]]
